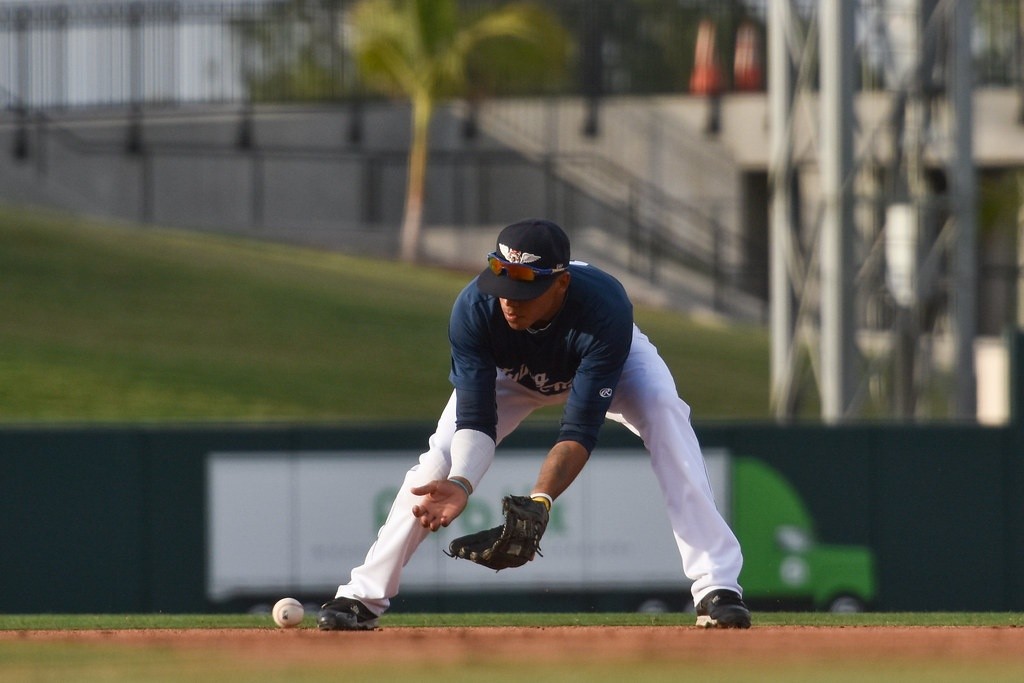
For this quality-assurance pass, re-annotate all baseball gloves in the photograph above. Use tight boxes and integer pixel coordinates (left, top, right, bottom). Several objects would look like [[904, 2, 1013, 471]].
[[442, 495, 551, 570]]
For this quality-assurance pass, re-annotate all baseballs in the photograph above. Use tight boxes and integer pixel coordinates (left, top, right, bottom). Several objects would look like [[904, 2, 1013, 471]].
[[272, 597, 304, 627]]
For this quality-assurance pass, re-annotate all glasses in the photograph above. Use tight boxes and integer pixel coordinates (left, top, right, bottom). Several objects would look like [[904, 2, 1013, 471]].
[[487, 250, 570, 282]]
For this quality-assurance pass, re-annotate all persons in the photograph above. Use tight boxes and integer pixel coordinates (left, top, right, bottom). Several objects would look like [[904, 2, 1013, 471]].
[[316, 220, 751, 631]]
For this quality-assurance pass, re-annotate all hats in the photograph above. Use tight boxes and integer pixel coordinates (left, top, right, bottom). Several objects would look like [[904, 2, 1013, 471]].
[[475, 219, 571, 299]]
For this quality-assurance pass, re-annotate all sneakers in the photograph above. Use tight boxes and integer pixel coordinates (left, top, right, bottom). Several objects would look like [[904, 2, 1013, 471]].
[[695, 589, 751, 629], [318, 597, 378, 630]]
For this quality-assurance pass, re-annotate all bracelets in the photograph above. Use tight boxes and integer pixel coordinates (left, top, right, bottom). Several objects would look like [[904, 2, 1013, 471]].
[[529, 493, 553, 511], [447, 479, 470, 496]]
